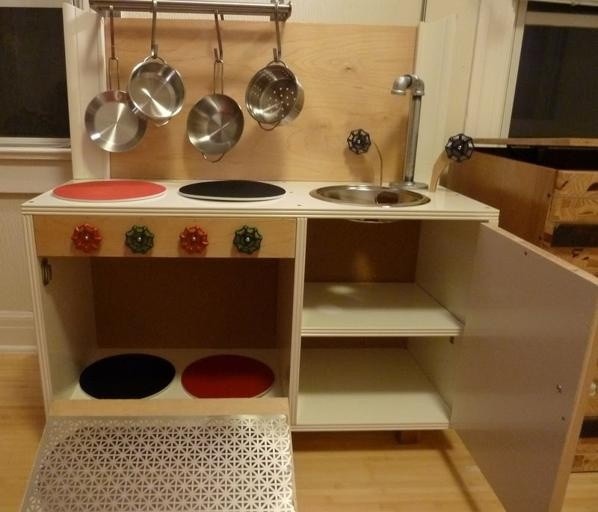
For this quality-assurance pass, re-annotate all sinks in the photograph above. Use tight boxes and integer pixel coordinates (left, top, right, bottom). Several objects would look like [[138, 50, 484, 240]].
[[310, 183, 431, 207]]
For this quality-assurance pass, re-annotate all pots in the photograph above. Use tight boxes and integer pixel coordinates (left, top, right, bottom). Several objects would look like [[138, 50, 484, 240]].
[[86, 3, 304, 167]]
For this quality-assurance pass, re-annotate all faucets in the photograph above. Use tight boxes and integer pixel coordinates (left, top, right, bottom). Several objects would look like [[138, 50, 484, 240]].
[[391, 73, 425, 189]]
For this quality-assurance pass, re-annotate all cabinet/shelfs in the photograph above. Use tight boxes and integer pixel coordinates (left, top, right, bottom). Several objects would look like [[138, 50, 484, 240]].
[[290, 205, 598, 511]]
